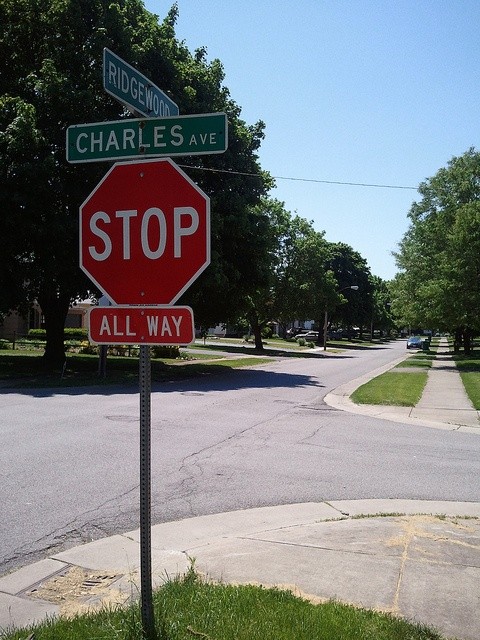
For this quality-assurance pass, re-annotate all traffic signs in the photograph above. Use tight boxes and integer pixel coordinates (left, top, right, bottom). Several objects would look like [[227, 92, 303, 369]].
[[79, 158, 211, 308]]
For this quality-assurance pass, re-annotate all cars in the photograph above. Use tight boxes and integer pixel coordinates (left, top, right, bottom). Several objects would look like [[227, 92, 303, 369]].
[[334, 329, 358, 338], [407, 337, 423, 349], [296, 331, 319, 341]]
